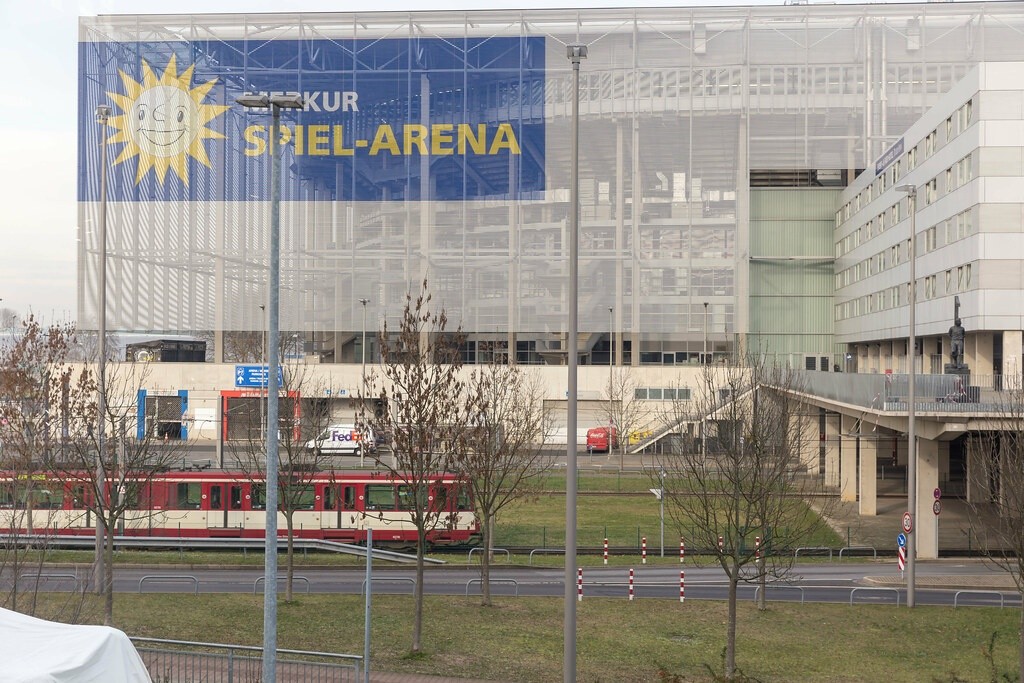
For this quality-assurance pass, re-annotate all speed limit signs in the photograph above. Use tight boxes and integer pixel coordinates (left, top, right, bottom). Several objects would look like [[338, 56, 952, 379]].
[[933, 500, 941, 515], [902, 512, 913, 534]]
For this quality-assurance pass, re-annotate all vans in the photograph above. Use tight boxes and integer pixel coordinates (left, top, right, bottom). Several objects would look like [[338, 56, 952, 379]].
[[306, 423, 376, 456]]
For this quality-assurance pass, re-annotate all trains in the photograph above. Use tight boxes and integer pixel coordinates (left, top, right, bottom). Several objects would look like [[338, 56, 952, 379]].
[[1, 463, 484, 551]]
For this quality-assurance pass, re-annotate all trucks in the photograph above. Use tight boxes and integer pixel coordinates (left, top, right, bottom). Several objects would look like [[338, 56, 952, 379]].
[[586, 428, 619, 452]]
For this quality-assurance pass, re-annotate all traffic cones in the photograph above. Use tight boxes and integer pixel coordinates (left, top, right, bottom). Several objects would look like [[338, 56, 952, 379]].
[[164, 432, 169, 444]]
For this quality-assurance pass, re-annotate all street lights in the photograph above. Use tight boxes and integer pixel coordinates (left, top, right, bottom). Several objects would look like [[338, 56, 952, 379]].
[[258, 304, 266, 452], [94, 100, 113, 594], [606, 306, 615, 461], [12, 315, 16, 367], [703, 301, 708, 475], [895, 182, 922, 607], [239, 92, 305, 683], [357, 297, 370, 468], [566, 42, 589, 682]]
